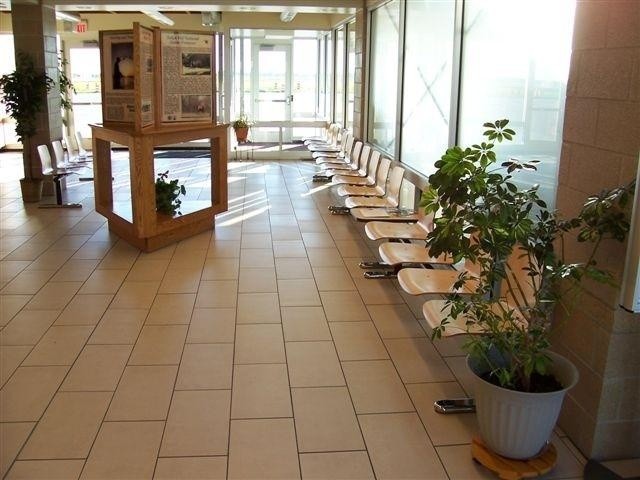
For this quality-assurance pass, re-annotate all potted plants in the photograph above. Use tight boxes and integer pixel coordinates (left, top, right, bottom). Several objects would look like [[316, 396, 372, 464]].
[[156, 170, 187, 217], [0, 50, 55, 202], [232, 116, 251, 144], [419, 120, 635, 460]]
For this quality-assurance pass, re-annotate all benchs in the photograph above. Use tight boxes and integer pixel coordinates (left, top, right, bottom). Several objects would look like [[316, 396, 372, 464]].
[[365, 204, 543, 414], [304, 125, 416, 269]]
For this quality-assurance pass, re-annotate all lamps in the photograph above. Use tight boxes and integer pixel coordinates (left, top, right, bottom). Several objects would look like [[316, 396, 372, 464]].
[[57, 12, 82, 25], [36, 131, 115, 208], [142, 11, 175, 27]]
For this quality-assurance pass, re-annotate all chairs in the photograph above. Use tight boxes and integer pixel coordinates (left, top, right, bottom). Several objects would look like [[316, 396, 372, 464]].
[[36, 131, 115, 208]]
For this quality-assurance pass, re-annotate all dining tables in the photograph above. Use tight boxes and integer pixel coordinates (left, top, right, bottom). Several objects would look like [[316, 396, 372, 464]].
[[419, 120, 635, 460], [232, 116, 251, 144], [0, 50, 55, 202], [156, 170, 187, 217]]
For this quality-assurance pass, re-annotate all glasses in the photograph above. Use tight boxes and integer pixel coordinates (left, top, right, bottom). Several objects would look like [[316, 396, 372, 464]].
[[57, 12, 82, 25], [142, 11, 175, 27]]
